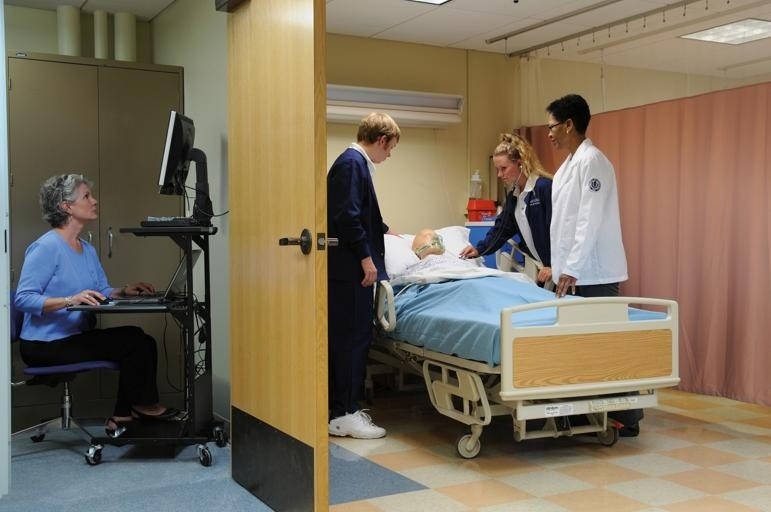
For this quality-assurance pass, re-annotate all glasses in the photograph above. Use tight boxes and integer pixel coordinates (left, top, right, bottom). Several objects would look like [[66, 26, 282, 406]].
[[547, 122, 563, 130]]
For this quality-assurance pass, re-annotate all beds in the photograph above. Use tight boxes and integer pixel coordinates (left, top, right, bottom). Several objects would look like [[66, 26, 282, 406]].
[[369, 226, 680, 459]]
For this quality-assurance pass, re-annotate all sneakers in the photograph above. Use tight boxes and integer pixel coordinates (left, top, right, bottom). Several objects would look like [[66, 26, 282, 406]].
[[329, 410, 385, 438]]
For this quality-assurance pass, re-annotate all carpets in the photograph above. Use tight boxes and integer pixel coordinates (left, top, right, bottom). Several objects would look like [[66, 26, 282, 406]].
[[0, 415, 430, 512]]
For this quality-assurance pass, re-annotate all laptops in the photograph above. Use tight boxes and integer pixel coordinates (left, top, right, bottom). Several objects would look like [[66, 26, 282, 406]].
[[112, 249, 202, 306]]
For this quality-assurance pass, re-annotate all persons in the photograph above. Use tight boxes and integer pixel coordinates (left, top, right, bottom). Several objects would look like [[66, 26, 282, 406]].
[[13, 172, 180, 438], [412, 227, 446, 259], [546, 94, 645, 437], [327, 109, 403, 441], [459, 131, 555, 290]]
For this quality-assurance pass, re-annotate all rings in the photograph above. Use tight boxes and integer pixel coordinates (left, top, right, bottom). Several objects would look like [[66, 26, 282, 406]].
[[86, 295, 92, 299]]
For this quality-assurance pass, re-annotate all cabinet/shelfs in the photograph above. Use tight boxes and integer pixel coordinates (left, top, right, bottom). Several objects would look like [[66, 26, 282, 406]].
[[7, 54, 187, 434]]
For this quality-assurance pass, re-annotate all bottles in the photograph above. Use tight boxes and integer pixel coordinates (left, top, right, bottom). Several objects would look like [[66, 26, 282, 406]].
[[469, 170, 483, 199]]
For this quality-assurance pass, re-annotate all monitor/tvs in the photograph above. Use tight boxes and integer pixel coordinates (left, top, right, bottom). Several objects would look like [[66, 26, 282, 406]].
[[157, 112, 213, 228]]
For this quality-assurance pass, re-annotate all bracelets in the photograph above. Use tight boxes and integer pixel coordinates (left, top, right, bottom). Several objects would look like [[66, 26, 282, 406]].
[[65, 296, 73, 307], [121, 285, 128, 296]]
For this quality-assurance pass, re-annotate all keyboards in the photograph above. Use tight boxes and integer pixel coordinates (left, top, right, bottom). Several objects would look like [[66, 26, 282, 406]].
[[140, 215, 194, 228]]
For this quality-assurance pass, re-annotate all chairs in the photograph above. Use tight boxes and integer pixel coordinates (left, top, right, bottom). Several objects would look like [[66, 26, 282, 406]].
[[10, 289, 121, 466]]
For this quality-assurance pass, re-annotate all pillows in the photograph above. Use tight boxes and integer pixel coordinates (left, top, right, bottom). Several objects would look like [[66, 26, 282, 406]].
[[380, 227, 471, 278]]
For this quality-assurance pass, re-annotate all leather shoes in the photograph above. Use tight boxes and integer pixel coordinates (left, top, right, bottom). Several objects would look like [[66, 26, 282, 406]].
[[132, 406, 182, 419], [108, 417, 147, 434], [620, 423, 642, 435]]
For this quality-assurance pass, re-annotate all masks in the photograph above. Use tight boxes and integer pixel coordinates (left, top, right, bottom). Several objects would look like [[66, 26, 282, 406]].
[[418, 233, 444, 256]]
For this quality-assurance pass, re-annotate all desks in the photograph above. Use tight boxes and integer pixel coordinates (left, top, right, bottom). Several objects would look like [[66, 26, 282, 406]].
[[67, 226, 225, 467]]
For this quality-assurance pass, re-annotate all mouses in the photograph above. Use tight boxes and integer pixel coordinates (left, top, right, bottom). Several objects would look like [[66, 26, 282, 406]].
[[82, 297, 109, 304]]
